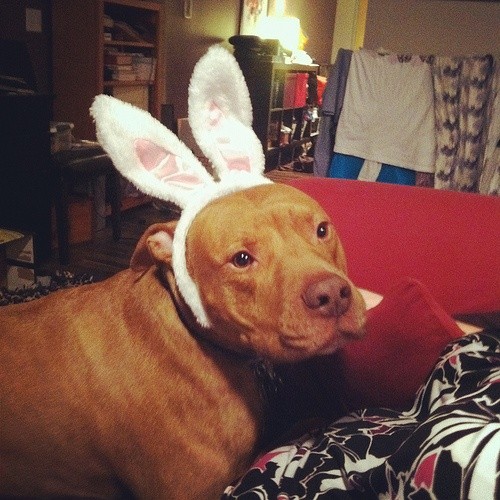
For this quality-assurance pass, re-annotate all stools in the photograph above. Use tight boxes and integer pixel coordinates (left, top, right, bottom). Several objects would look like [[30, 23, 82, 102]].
[[48, 138, 122, 265]]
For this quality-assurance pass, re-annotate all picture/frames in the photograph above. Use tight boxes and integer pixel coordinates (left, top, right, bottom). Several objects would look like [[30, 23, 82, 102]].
[[235, 1, 269, 39]]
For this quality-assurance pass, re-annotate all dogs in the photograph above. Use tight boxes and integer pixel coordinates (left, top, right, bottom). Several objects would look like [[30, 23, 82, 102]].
[[1, 183, 367, 499]]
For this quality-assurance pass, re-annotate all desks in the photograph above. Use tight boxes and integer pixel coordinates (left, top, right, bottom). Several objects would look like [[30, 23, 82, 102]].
[[1, 92, 58, 276]]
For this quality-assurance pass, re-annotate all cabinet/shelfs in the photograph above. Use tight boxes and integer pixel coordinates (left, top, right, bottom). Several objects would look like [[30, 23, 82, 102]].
[[237, 61, 320, 174], [50, 0, 167, 217]]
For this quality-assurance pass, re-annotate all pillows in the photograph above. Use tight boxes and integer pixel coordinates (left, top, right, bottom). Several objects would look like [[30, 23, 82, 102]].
[[272, 176, 500, 411]]
